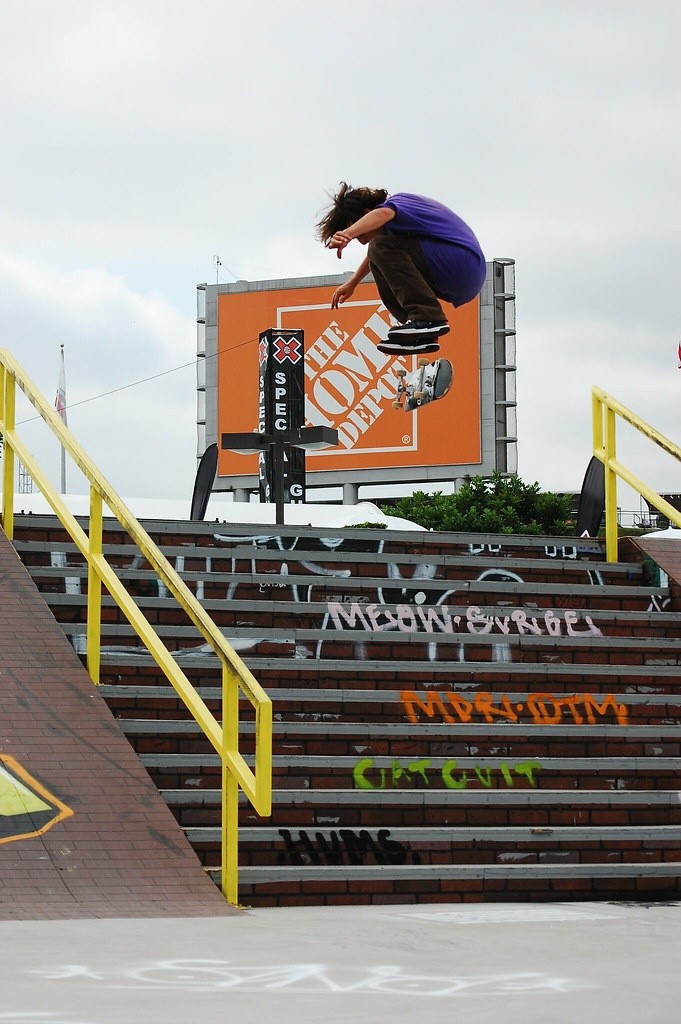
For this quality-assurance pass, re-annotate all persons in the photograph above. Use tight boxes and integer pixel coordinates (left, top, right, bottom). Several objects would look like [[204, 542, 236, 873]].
[[314, 180, 487, 356]]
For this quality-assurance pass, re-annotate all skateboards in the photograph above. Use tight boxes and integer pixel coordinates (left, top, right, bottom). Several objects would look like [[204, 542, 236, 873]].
[[391, 358, 455, 411]]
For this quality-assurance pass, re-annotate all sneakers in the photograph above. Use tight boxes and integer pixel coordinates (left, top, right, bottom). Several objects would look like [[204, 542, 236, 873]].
[[377, 338, 440, 355], [387, 318, 451, 342]]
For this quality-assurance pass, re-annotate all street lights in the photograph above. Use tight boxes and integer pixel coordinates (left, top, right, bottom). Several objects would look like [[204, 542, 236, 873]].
[[221, 424, 340, 525]]
